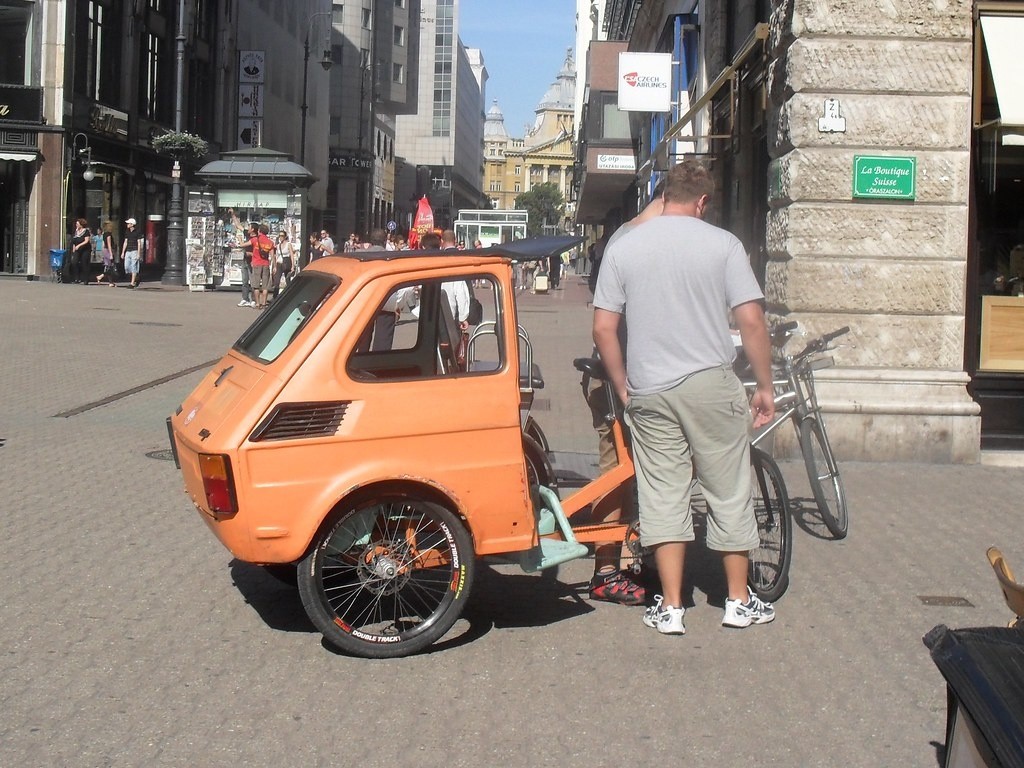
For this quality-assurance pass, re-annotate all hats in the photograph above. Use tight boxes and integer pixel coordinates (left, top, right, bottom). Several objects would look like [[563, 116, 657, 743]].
[[125, 218, 136, 225]]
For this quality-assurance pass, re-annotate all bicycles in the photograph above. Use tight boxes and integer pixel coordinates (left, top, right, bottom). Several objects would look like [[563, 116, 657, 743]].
[[733, 320, 851, 540]]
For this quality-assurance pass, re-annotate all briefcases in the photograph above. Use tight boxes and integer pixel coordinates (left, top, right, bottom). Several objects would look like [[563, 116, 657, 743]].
[[535, 271, 549, 291]]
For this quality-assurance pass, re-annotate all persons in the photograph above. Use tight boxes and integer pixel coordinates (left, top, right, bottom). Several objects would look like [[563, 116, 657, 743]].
[[592, 159, 775, 633], [354, 229, 401, 352], [583, 178, 667, 607], [121, 218, 141, 289], [411, 233, 471, 353], [227, 206, 295, 309], [441, 230, 475, 300], [70, 219, 117, 288], [306, 228, 579, 295]]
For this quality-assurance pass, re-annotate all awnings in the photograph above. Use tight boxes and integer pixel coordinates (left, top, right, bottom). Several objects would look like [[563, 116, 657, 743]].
[[0, 145, 41, 162], [974, 16, 1024, 145]]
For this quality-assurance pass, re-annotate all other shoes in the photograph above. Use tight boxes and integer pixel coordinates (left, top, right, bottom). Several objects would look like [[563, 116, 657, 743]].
[[109, 284, 116, 287], [127, 281, 141, 289], [237, 299, 266, 309], [96, 275, 99, 284], [72, 280, 81, 284], [84, 281, 88, 285]]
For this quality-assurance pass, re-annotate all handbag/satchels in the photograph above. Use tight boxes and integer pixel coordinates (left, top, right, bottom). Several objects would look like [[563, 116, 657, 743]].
[[467, 297, 482, 324], [257, 235, 270, 259], [281, 257, 292, 272], [457, 328, 470, 365]]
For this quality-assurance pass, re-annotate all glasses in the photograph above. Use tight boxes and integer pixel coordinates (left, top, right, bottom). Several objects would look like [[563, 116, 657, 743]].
[[279, 235, 284, 237]]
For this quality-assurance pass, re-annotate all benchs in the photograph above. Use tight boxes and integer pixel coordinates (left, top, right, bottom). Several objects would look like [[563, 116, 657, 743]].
[[437, 289, 545, 389]]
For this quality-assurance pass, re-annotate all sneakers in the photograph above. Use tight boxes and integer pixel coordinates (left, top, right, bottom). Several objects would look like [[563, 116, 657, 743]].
[[643, 594, 687, 634], [721, 587, 775, 627], [590, 571, 654, 606]]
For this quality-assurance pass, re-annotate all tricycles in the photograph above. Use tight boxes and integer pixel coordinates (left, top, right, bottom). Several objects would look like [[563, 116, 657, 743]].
[[166, 234, 794, 659]]
